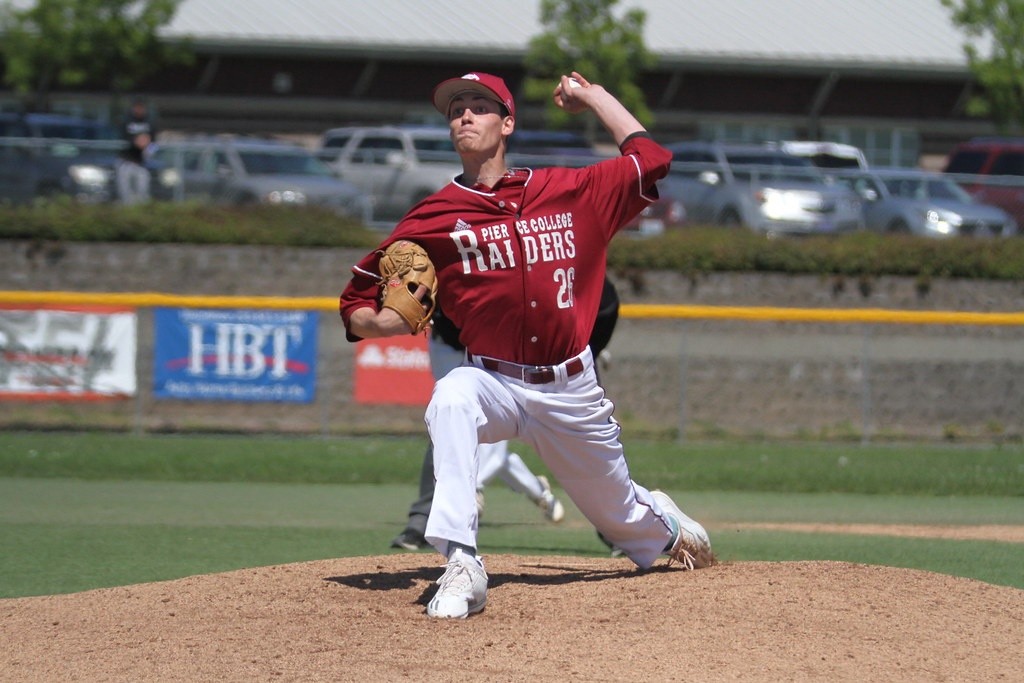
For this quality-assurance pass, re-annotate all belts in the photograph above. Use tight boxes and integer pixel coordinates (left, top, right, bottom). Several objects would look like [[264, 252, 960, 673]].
[[468, 351, 583, 384]]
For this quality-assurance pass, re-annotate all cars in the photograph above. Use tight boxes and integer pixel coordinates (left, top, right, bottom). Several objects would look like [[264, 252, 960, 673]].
[[653, 141, 864, 234], [505, 130, 604, 167], [834, 169, 1019, 238], [157, 140, 373, 224], [944, 137, 1024, 234], [0, 111, 177, 203], [319, 127, 464, 217]]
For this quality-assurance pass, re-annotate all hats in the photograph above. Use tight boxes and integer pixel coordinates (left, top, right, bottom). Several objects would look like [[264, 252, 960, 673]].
[[432, 72, 515, 120]]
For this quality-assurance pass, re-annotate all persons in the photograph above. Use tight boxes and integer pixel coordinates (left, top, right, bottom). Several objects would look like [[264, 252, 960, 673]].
[[340, 71, 714, 616], [108, 120, 158, 206]]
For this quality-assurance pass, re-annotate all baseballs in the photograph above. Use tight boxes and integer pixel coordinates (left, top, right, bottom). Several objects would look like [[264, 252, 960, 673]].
[[558, 76, 582, 88]]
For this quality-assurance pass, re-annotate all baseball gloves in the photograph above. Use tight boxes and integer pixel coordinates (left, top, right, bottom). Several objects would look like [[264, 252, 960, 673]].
[[378, 240, 439, 334]]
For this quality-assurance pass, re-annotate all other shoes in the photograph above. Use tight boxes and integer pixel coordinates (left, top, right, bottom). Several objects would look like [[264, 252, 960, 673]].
[[392, 528, 425, 550]]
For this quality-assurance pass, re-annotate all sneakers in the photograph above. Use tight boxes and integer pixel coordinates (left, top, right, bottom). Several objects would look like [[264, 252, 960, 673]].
[[650, 491, 712, 571], [427, 546, 489, 620], [532, 475, 564, 523]]
